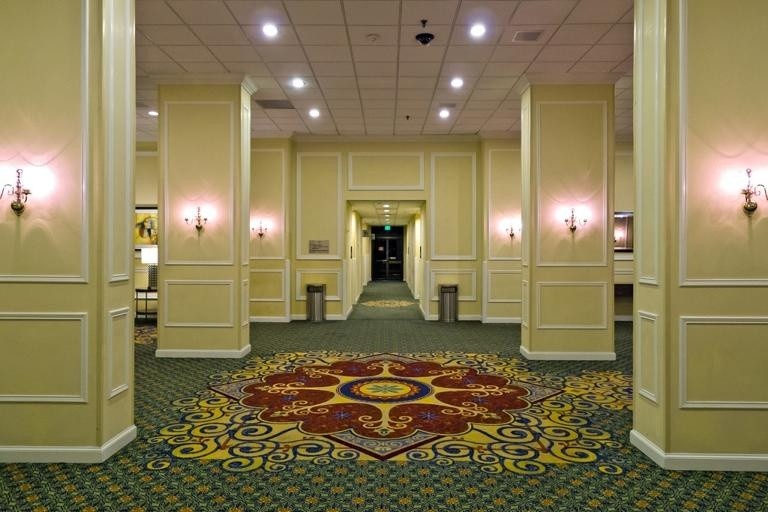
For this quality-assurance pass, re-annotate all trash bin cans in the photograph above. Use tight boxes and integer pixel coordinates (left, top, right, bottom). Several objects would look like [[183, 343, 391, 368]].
[[306, 283, 326, 322], [439, 284, 458, 323]]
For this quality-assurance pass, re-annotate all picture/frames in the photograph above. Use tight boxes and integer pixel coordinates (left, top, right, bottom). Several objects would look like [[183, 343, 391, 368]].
[[133, 205, 159, 251]]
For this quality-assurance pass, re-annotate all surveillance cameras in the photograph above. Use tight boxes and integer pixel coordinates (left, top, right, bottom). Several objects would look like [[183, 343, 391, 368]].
[[413, 32, 436, 47]]
